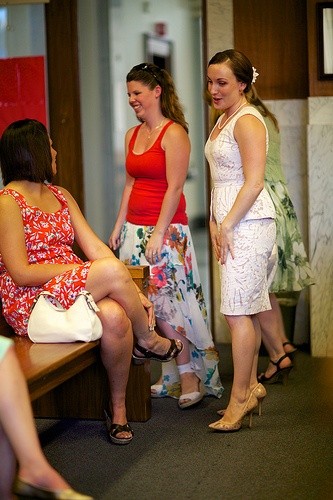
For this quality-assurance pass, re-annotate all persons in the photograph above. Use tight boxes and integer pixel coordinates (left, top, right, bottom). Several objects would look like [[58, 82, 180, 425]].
[[204, 78, 316, 387], [108, 63, 225, 409], [204, 49, 277, 431], [0, 336, 93, 500], [0, 118, 184, 445]]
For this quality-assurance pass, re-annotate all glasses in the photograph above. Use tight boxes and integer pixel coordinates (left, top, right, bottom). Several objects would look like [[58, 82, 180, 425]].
[[133, 64, 162, 85]]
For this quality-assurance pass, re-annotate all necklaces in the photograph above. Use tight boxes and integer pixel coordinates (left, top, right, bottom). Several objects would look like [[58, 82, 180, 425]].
[[146, 120, 164, 139], [218, 101, 246, 129]]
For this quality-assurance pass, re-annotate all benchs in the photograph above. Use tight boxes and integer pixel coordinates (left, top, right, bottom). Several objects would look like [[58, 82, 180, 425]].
[[10, 266, 153, 421]]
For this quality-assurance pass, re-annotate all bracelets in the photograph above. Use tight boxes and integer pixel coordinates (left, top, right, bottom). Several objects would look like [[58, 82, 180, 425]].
[[138, 291, 146, 295]]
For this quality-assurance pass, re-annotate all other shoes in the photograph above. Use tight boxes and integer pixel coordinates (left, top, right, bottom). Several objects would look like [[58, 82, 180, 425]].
[[11, 477, 94, 500]]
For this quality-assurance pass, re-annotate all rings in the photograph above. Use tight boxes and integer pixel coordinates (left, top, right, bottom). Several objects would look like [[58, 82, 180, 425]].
[[152, 253, 156, 255]]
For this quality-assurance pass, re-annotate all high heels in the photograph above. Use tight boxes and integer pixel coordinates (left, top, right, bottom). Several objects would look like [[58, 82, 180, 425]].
[[177, 365, 206, 408], [209, 392, 258, 432], [216, 383, 267, 416], [106, 403, 134, 444], [282, 342, 297, 369], [132, 338, 184, 367], [151, 359, 177, 397], [258, 354, 294, 386]]
